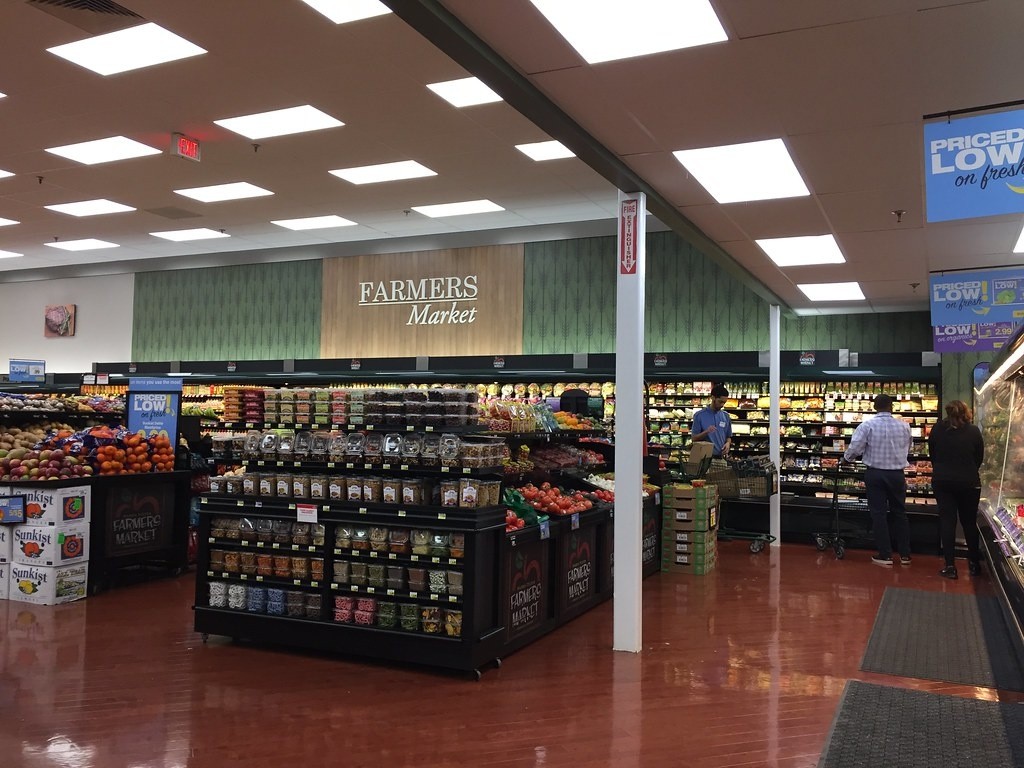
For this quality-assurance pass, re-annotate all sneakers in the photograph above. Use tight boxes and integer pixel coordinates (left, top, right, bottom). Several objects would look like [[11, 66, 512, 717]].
[[872, 554, 894, 564], [900, 554, 912, 564]]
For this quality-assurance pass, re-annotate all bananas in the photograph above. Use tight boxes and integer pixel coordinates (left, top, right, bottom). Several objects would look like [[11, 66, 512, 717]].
[[182, 404, 215, 416]]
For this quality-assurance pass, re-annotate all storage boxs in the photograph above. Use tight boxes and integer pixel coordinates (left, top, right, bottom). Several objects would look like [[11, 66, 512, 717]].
[[660, 441, 778, 576], [207, 528, 464, 637], [0, 486, 92, 606], [220, 385, 482, 429], [702, 382, 714, 393], [693, 381, 702, 393]]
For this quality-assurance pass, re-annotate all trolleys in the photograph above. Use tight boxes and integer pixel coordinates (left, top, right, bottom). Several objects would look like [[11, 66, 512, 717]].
[[806, 463, 877, 560], [673, 453, 777, 553]]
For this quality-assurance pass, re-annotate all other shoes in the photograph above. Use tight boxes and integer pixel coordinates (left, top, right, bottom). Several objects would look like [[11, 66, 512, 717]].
[[969, 560, 980, 575], [941, 566, 957, 578]]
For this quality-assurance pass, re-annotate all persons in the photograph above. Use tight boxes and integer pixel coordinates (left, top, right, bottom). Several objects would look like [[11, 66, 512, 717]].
[[928, 400, 985, 580], [838, 393, 913, 565], [691, 384, 732, 474]]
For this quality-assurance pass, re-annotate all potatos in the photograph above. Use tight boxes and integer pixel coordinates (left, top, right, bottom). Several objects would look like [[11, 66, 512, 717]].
[[0, 419, 105, 450], [0, 396, 66, 413]]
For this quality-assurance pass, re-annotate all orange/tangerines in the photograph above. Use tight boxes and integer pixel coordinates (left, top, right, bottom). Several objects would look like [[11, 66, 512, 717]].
[[553, 410, 583, 430], [40, 426, 175, 476]]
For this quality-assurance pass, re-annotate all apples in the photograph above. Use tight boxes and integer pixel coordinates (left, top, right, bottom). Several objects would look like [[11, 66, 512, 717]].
[[0, 448, 93, 481]]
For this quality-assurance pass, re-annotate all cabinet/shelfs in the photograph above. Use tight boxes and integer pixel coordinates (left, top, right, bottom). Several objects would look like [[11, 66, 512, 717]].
[[189, 421, 506, 683], [605, 393, 939, 516]]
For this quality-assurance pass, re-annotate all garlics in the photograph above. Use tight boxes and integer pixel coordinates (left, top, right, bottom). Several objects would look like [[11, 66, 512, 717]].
[[585, 474, 650, 497]]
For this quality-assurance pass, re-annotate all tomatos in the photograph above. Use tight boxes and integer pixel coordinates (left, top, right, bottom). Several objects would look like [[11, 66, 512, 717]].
[[515, 482, 614, 515], [504, 509, 525, 532]]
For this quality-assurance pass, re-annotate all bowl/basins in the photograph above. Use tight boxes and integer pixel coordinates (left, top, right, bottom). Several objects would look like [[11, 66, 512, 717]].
[[221, 386, 479, 435], [208, 518, 464, 637]]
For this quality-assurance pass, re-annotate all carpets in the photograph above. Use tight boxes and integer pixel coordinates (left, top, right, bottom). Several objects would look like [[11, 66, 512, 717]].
[[858, 584, 1024, 693], [816, 677, 1024, 768]]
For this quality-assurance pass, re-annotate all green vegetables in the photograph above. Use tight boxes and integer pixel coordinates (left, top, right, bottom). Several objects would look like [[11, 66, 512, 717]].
[[978, 408, 1013, 486]]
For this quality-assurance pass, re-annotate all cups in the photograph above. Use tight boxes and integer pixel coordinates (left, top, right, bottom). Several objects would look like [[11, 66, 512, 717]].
[[212, 435, 505, 509]]
